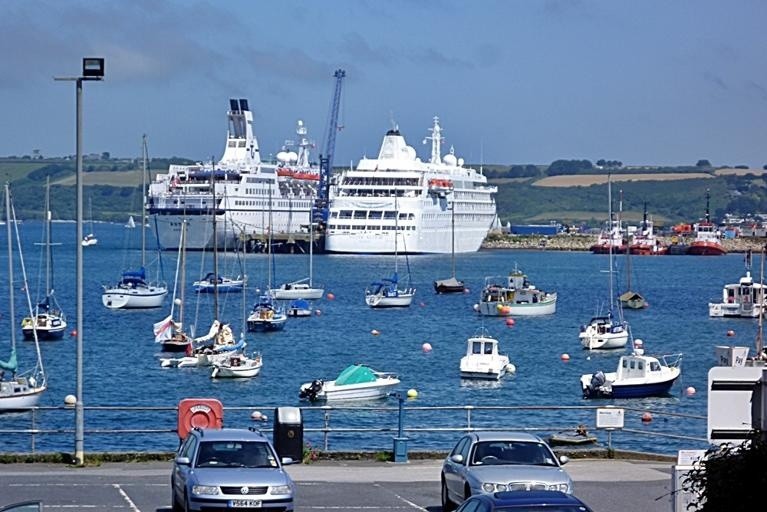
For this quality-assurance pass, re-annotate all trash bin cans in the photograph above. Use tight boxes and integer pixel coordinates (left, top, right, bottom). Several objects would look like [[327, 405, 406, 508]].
[[273, 407, 303, 463]]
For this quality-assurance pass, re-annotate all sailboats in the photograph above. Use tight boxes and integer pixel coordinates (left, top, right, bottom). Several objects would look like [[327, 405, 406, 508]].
[[707, 242, 767, 367], [364, 186, 416, 308], [577, 170, 630, 350], [433, 200, 464, 294], [616, 222, 646, 309]]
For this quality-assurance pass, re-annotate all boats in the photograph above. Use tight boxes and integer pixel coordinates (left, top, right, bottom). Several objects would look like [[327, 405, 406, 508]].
[[579, 338, 683, 399], [479, 262, 558, 316], [583, 346, 630, 359], [707, 245, 767, 317], [298, 362, 400, 403], [580, 393, 680, 408], [460, 377, 502, 390], [588, 221, 726, 255], [459, 320, 510, 380]]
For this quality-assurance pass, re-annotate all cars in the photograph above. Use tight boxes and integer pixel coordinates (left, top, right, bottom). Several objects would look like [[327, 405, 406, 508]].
[[441, 432, 595, 512], [170, 426, 293, 512]]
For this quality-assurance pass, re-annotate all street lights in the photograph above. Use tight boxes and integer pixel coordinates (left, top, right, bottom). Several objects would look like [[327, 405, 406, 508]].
[[54, 59, 106, 468]]
